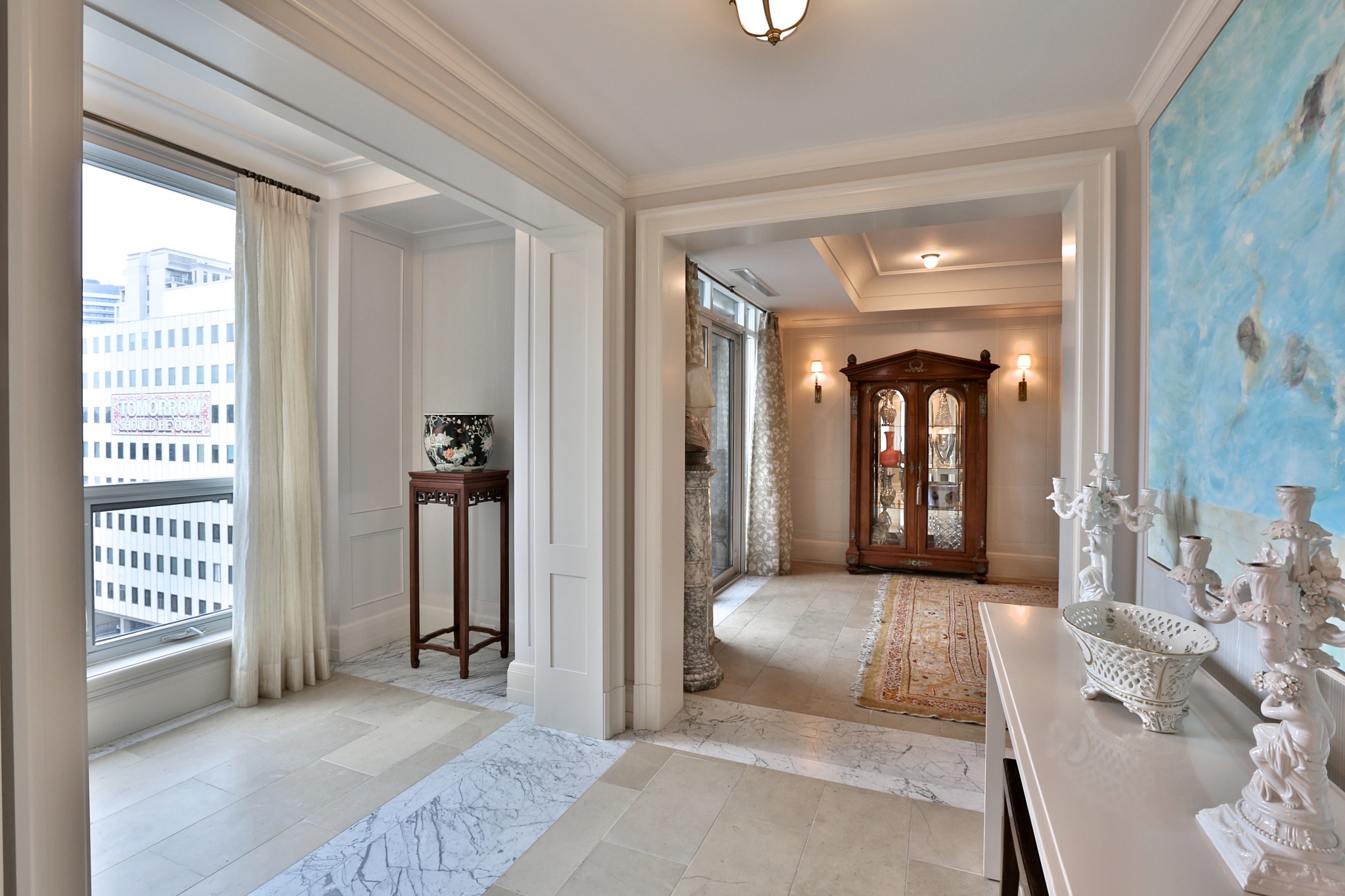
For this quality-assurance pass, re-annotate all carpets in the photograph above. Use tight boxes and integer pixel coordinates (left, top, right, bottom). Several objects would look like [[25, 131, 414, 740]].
[[847, 573, 1058, 729]]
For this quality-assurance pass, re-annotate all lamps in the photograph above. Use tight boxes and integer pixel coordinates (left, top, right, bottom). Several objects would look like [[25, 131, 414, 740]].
[[728, 0, 810, 46], [921, 253, 941, 270], [1016, 354, 1031, 402], [810, 361, 824, 403]]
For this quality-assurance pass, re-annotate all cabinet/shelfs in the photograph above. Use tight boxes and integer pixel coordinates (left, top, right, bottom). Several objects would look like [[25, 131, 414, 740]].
[[839, 348, 1002, 585]]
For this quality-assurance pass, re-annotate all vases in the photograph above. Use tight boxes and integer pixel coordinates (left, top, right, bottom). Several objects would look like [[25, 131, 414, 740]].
[[424, 412, 495, 472], [880, 430, 898, 467], [880, 388, 897, 427], [934, 388, 953, 425], [880, 476, 894, 507]]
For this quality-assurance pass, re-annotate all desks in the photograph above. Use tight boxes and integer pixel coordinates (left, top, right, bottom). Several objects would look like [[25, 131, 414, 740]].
[[978, 599, 1345, 896], [407, 469, 510, 679]]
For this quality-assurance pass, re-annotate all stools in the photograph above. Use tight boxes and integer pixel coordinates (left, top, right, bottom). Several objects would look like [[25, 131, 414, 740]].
[[1000, 757, 1050, 896]]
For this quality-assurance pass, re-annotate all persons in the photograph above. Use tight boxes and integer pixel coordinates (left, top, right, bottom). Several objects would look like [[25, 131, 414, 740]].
[[1079, 535, 1115, 596], [1249, 661, 1326, 807]]
